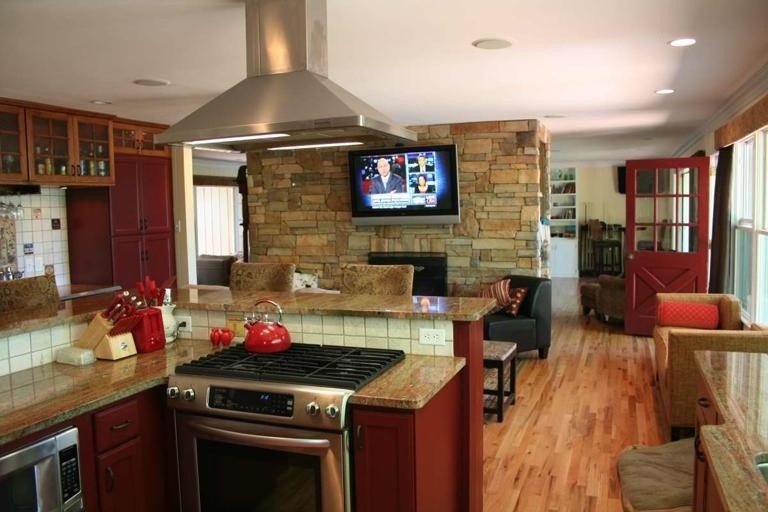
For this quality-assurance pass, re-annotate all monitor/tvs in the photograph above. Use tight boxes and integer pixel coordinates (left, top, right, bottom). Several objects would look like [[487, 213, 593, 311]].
[[347, 144, 461, 227]]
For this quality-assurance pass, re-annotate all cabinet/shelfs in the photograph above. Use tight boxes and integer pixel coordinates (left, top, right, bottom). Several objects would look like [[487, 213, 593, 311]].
[[78, 384, 167, 512], [348, 355, 467, 512], [26, 100, 116, 186], [0, 96, 29, 185], [691, 349, 768, 512], [550, 166, 580, 278], [66, 153, 178, 303]]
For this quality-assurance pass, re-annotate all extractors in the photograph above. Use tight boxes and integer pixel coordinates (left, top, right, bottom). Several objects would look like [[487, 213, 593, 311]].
[[154, 0, 418, 151]]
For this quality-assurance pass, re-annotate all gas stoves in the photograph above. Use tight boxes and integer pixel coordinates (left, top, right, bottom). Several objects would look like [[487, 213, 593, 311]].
[[166, 341, 406, 431]]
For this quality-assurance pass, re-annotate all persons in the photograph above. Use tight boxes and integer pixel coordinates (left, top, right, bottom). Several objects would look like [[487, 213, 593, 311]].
[[370, 158, 403, 197], [412, 155, 434, 173], [415, 175, 433, 193]]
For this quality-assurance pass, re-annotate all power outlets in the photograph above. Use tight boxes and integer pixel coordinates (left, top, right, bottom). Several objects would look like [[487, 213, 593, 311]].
[[175, 316, 192, 332], [419, 328, 446, 346]]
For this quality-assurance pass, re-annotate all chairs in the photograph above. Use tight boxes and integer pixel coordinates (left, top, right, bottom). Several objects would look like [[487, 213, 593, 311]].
[[0, 272, 60, 314], [229, 261, 296, 292], [339, 262, 416, 297]]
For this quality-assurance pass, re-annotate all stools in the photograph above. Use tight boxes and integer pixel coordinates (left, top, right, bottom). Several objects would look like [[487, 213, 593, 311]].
[[484, 340, 518, 423]]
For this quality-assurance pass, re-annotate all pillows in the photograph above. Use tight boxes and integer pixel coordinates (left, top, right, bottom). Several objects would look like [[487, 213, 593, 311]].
[[478, 277, 512, 317], [656, 300, 719, 329], [500, 287, 529, 317]]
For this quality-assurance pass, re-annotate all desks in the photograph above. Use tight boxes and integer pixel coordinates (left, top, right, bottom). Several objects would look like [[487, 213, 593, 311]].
[[592, 239, 622, 275], [57, 283, 122, 301]]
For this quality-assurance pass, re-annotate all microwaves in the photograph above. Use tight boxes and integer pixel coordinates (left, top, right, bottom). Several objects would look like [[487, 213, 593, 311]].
[[1, 425, 86, 512]]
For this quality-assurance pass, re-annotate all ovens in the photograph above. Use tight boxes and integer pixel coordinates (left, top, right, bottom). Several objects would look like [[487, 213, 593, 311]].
[[167, 411, 353, 511]]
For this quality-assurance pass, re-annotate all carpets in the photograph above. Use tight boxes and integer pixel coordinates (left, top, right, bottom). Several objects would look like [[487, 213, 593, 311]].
[[483, 353, 529, 427]]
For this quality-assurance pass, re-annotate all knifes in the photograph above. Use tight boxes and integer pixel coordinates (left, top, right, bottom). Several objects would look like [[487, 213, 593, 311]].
[[73, 290, 144, 360]]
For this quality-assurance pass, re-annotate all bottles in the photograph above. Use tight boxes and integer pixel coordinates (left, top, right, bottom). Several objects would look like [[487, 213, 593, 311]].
[[88, 142, 96, 176], [44, 147, 51, 175], [97, 145, 106, 176], [35, 144, 42, 174], [80, 159, 88, 176]]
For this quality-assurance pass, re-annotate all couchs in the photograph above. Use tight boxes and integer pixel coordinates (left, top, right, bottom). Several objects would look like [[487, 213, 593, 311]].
[[580, 273, 625, 324], [652, 292, 768, 442], [484, 274, 553, 358]]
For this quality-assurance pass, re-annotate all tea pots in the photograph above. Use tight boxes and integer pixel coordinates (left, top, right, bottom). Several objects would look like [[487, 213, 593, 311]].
[[244, 299, 291, 353]]
